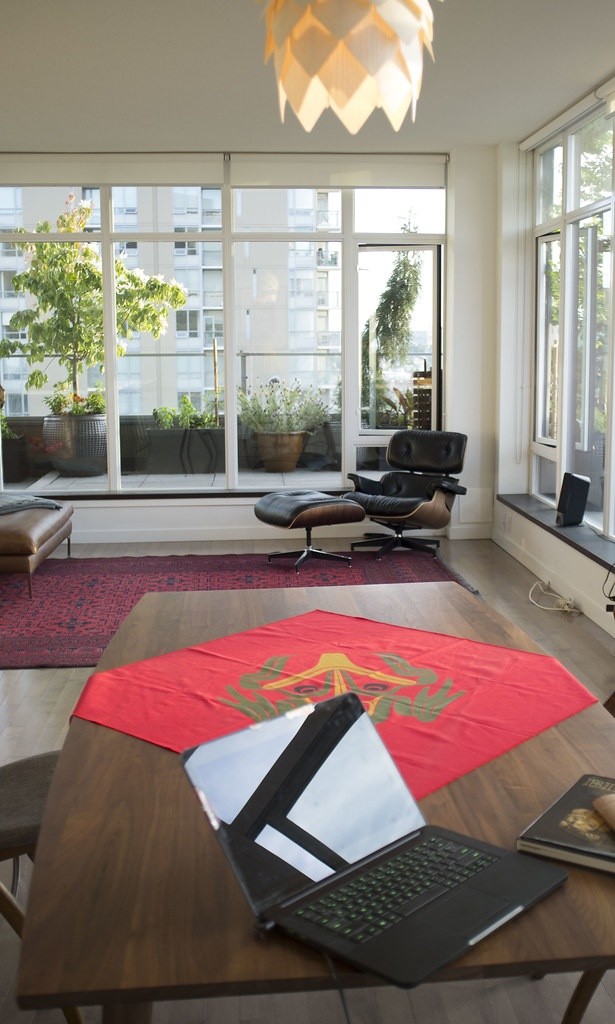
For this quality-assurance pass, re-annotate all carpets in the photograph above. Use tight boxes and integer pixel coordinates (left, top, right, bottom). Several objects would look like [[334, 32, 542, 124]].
[[0, 548, 459, 669]]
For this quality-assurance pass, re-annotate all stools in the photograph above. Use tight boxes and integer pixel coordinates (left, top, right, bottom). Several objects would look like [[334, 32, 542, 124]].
[[253, 488, 368, 576], [0, 491, 74, 600]]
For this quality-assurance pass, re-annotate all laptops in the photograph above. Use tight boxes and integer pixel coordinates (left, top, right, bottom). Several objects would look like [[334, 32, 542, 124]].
[[179, 690, 570, 991]]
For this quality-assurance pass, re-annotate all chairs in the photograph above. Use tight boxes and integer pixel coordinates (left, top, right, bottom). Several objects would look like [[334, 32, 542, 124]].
[[0, 748, 88, 1023], [337, 429, 468, 564]]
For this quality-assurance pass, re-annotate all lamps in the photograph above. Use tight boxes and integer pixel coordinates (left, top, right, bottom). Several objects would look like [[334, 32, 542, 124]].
[[262, 0, 436, 137]]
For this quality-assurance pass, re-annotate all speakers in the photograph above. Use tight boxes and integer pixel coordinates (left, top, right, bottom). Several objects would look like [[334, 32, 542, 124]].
[[555, 472, 590, 527]]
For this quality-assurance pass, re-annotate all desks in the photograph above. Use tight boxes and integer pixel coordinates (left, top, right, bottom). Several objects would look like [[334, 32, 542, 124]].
[[10, 580, 615, 1024]]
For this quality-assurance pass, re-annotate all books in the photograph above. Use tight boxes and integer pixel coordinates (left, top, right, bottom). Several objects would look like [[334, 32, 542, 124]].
[[516, 771, 615, 872]]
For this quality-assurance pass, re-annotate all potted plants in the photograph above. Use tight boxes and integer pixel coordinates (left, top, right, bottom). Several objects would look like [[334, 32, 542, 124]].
[[373, 386, 417, 472], [0, 193, 190, 480], [236, 375, 337, 473], [1, 407, 32, 483], [322, 208, 424, 471], [143, 385, 226, 474]]
